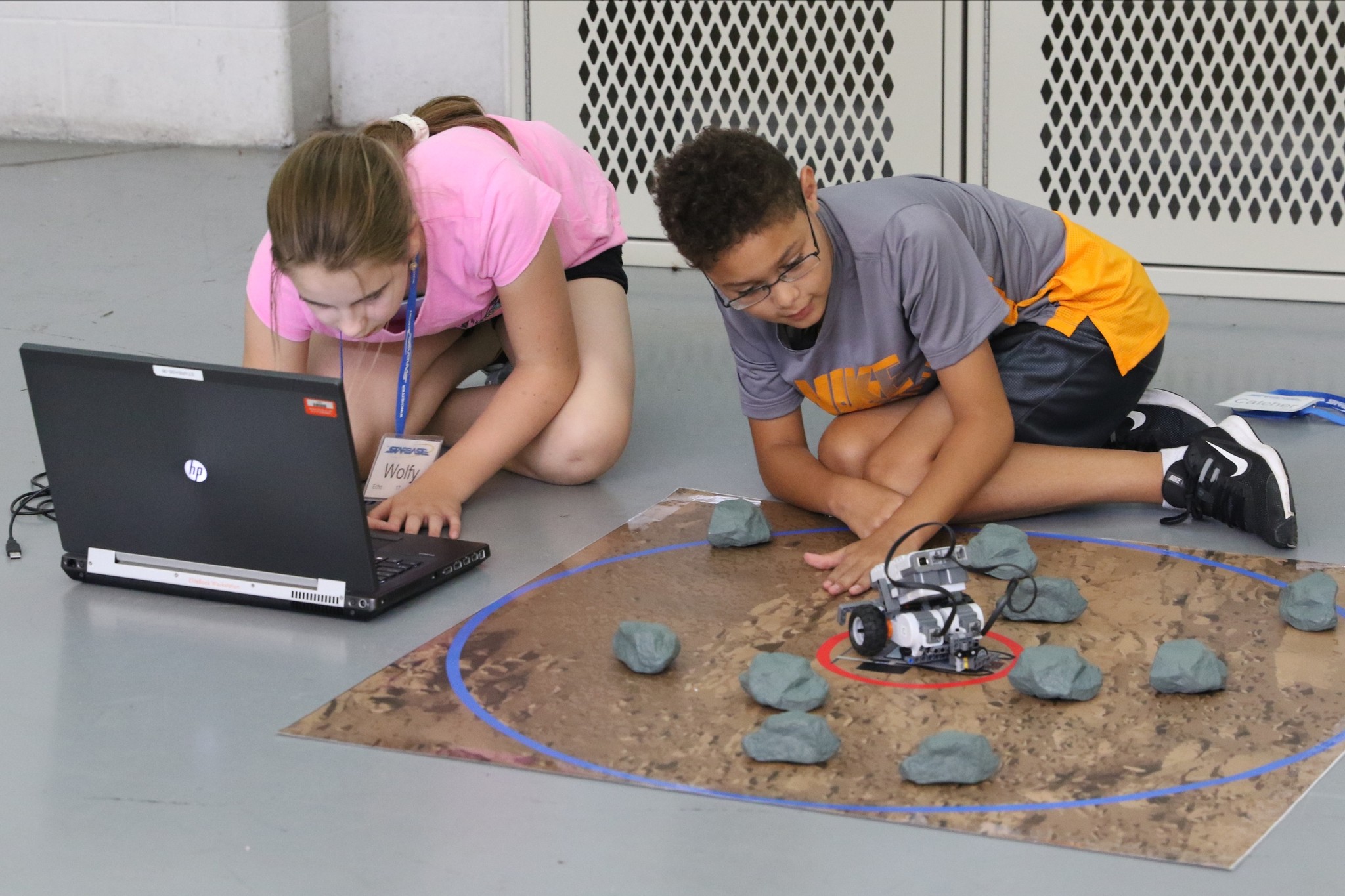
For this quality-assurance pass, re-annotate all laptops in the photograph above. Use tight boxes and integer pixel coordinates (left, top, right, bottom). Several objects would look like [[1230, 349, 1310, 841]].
[[19, 342, 491, 616]]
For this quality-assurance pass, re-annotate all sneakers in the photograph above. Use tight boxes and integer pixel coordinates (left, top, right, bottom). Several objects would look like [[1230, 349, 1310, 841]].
[[1160, 415, 1298, 548], [480, 361, 514, 386], [1101, 387, 1217, 452]]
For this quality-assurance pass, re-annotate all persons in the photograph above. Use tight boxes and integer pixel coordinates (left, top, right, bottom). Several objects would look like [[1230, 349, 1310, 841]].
[[243, 95, 637, 539], [654, 127, 1298, 595]]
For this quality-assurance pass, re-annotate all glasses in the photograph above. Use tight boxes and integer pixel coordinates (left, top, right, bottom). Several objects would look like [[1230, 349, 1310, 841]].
[[701, 181, 820, 310]]
